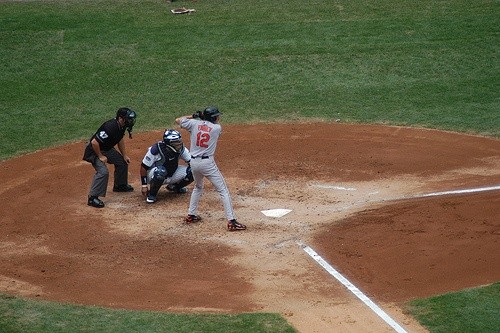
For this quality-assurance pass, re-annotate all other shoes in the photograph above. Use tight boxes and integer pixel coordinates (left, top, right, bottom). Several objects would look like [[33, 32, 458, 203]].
[[227, 219, 246, 231], [112, 185, 132, 191], [87, 195, 104, 208], [166, 184, 188, 194], [187, 215, 201, 223], [145, 194, 156, 204]]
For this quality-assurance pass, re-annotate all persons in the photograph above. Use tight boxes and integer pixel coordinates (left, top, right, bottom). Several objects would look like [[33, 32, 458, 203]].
[[175, 107, 247, 230], [83, 107, 137, 207], [140, 128, 195, 203]]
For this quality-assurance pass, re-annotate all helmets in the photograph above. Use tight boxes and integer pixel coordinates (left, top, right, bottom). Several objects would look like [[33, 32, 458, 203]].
[[203, 106, 221, 116], [116, 107, 137, 132], [163, 129, 181, 142]]
